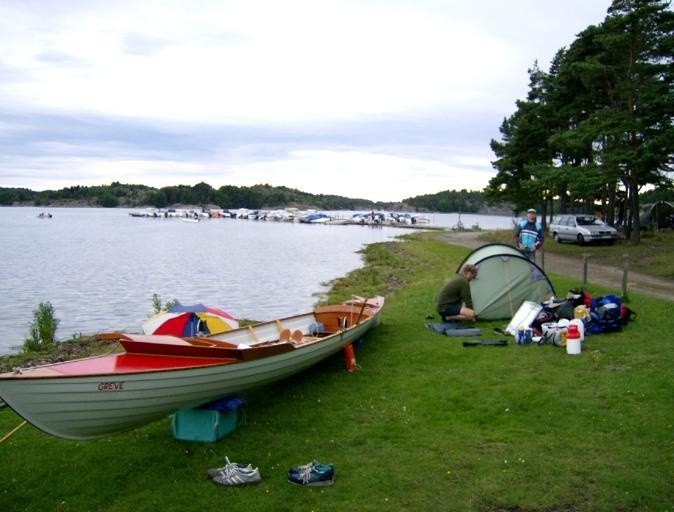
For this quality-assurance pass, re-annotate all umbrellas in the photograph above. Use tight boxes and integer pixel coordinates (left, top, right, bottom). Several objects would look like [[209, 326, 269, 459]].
[[140, 301, 238, 338]]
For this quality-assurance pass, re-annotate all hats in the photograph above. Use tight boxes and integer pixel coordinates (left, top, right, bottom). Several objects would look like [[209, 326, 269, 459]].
[[527, 207, 537, 214]]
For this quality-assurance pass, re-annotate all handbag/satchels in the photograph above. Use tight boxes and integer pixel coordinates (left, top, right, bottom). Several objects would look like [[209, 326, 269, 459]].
[[537, 322, 568, 347]]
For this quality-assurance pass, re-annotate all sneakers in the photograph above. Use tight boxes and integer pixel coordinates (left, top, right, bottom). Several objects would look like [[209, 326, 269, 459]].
[[288, 460, 335, 477], [211, 466, 261, 486], [207, 455, 252, 478], [287, 466, 335, 487]]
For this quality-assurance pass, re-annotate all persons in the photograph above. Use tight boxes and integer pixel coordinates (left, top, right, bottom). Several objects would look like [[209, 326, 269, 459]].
[[434, 262, 476, 322], [514, 208, 546, 262]]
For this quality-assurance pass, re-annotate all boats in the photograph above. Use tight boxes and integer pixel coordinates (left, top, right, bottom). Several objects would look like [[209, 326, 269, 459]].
[[128, 206, 431, 227], [0, 295, 385, 443], [36, 215, 53, 219]]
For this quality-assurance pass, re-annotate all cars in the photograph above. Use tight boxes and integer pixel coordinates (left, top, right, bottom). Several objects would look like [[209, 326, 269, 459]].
[[549, 214, 620, 247]]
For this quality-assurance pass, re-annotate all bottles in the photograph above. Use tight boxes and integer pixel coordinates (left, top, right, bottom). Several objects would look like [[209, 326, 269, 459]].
[[565, 324, 580, 354], [517, 325, 524, 345], [525, 326, 531, 344]]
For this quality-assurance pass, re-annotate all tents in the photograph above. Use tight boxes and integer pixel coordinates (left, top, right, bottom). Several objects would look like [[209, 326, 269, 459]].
[[635, 200, 674, 230], [455, 242, 555, 322]]
